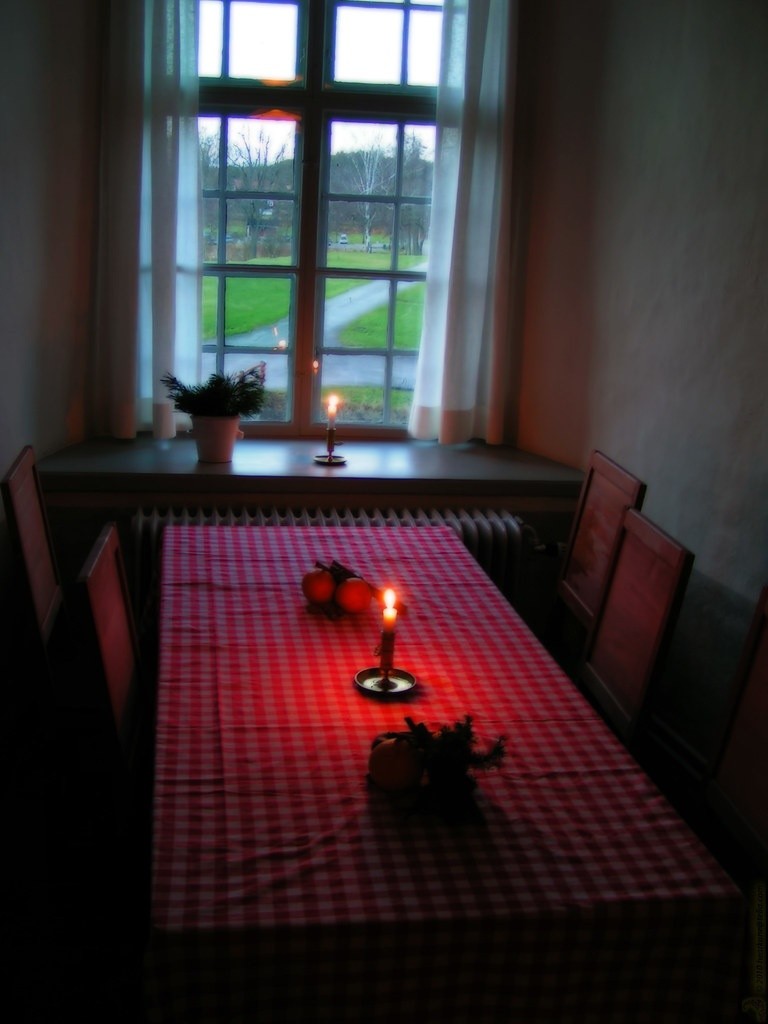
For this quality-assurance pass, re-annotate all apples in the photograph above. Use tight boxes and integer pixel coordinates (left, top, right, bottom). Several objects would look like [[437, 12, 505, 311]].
[[301, 570, 335, 605], [368, 738, 424, 788], [337, 577, 373, 614]]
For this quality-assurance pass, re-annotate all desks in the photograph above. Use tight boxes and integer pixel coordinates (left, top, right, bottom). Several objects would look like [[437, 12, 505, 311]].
[[150, 523, 748, 1023]]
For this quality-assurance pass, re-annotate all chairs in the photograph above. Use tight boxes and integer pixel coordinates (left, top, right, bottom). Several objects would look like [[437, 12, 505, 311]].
[[662, 578, 768, 1024], [0, 445, 118, 722], [533, 446, 648, 692], [76, 519, 154, 786], [570, 502, 696, 750]]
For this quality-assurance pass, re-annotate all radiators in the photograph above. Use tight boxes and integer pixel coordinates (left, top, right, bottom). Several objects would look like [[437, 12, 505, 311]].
[[126, 504, 543, 659]]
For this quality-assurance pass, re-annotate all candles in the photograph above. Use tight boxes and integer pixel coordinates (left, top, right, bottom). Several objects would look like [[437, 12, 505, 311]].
[[374, 587, 398, 630], [324, 393, 340, 428]]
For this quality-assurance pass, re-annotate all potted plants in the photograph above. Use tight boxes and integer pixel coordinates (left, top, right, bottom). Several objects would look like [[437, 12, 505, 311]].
[[159, 366, 267, 464]]
